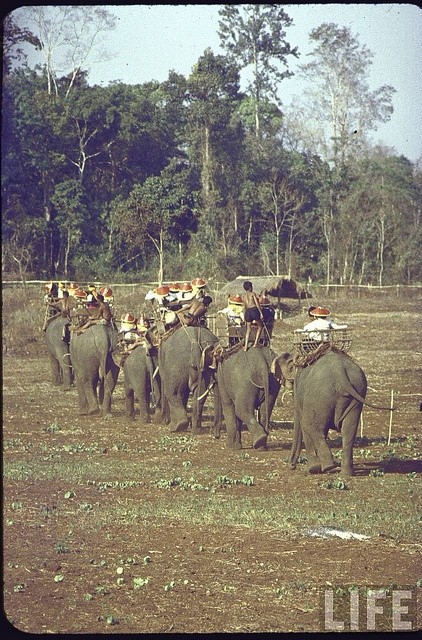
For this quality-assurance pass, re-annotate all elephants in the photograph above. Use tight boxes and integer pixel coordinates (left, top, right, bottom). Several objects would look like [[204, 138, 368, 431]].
[[40, 309, 81, 390], [61, 316, 125, 417], [198, 337, 282, 448], [112, 336, 166, 422], [269, 342, 397, 480], [146, 325, 224, 441]]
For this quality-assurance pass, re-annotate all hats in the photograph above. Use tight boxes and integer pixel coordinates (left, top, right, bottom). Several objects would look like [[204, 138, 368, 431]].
[[178, 283, 192, 292], [191, 277, 207, 287], [121, 313, 136, 323], [67, 283, 80, 291], [258, 294, 272, 306], [45, 280, 53, 292], [73, 290, 87, 297], [99, 287, 113, 298], [309, 306, 331, 317], [156, 285, 170, 296], [227, 294, 244, 305], [168, 283, 180, 292]]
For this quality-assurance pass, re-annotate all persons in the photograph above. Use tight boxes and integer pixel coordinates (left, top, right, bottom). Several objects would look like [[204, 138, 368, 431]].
[[144, 277, 212, 341], [120, 314, 149, 351], [44, 281, 80, 321], [71, 286, 114, 336], [217, 281, 275, 352], [292, 306, 348, 350]]
